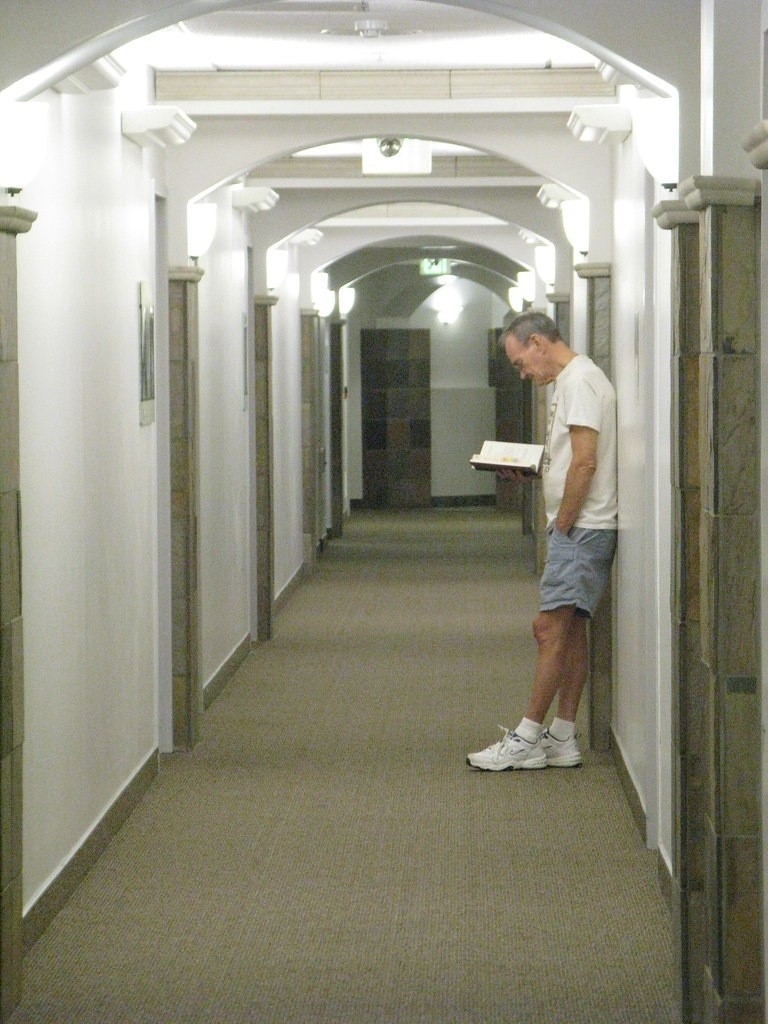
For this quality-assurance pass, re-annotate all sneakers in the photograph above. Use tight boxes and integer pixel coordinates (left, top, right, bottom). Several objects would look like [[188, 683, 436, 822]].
[[467, 723, 549, 772], [538, 730, 584, 768]]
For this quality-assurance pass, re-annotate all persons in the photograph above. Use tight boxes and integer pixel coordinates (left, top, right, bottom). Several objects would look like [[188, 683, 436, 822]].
[[466, 311, 620, 771]]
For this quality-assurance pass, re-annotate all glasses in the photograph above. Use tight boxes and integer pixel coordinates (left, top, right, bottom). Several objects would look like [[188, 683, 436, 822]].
[[511, 336, 531, 371]]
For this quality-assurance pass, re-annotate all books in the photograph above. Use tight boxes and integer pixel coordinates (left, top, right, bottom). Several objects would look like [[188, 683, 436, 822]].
[[469, 440, 544, 478]]
